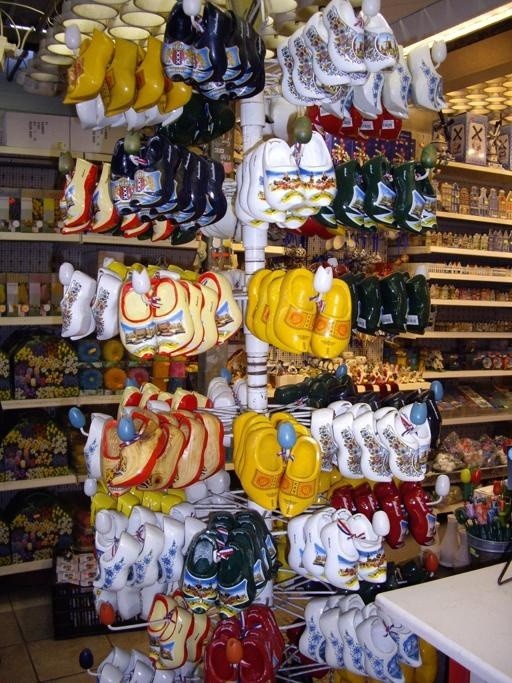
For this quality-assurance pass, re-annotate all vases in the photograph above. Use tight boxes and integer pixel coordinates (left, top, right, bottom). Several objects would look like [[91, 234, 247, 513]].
[[466, 531, 510, 567]]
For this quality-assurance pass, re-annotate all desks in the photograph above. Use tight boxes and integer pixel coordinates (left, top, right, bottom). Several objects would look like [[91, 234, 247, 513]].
[[374, 562, 512, 682]]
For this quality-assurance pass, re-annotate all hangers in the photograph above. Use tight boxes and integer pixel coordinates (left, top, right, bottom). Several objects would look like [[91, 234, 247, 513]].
[[59, 0, 448, 682]]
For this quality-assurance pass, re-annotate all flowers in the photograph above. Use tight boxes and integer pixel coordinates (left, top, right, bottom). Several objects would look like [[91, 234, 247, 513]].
[[455, 464, 511, 538]]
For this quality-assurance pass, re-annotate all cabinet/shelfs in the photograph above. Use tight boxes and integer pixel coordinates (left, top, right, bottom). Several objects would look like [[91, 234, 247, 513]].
[[0, 147, 511, 576]]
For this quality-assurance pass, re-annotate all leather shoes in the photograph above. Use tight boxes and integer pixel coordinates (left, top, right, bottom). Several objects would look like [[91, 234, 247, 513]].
[[344, 513, 388, 583], [321, 86, 352, 120], [116, 382, 212, 419], [152, 220, 171, 242], [93, 511, 125, 559], [355, 617, 405, 683], [137, 422, 185, 490], [382, 45, 412, 120], [302, 11, 350, 85], [274, 268, 317, 352], [380, 272, 410, 334], [287, 514, 321, 582], [399, 482, 423, 501], [322, 0, 366, 72], [314, 199, 337, 228], [248, 142, 287, 223], [299, 591, 327, 664], [262, 138, 306, 211], [58, 263, 97, 341], [193, 159, 227, 226], [207, 510, 280, 607], [436, 77, 448, 109], [152, 276, 195, 355], [351, 97, 378, 120], [170, 280, 205, 357], [170, 410, 207, 489], [126, 661, 153, 682], [185, 282, 219, 356], [120, 213, 143, 230], [83, 413, 113, 480], [91, 267, 122, 340], [130, 135, 180, 208], [311, 277, 352, 359], [184, 2, 227, 85], [198, 87, 229, 100], [193, 98, 235, 147], [160, 0, 196, 80], [205, 639, 238, 682], [199, 271, 243, 344], [398, 403, 431, 473], [97, 663, 122, 682], [245, 269, 273, 343], [350, 482, 372, 493], [154, 606, 193, 670], [186, 612, 210, 662], [392, 162, 423, 233], [97, 646, 128, 672], [230, 37, 266, 100], [124, 221, 152, 238], [275, 370, 421, 407], [241, 604, 286, 683], [221, 9, 248, 81], [129, 522, 164, 587], [241, 427, 284, 512], [276, 37, 316, 107], [198, 412, 226, 481], [406, 274, 430, 335], [310, 408, 339, 473], [406, 44, 440, 113], [63, 158, 97, 226], [295, 131, 338, 207], [303, 513, 329, 583], [333, 414, 365, 479], [206, 377, 248, 448], [109, 137, 141, 215], [91, 163, 120, 232], [364, 213, 379, 232], [160, 517, 186, 584], [172, 221, 200, 245], [333, 485, 353, 496], [212, 617, 241, 640], [361, 156, 397, 228], [101, 419, 132, 495], [162, 107, 183, 127], [378, 603, 422, 668], [232, 411, 310, 477], [346, 72, 369, 85], [153, 91, 194, 146], [151, 667, 177, 683], [226, 23, 259, 88], [172, 417, 205, 490], [405, 490, 438, 546], [353, 412, 393, 483], [374, 407, 397, 421], [374, 481, 399, 502], [321, 521, 361, 591], [357, 276, 380, 336], [422, 391, 442, 462], [68, 29, 113, 98], [118, 270, 160, 359], [261, 276, 303, 355], [327, 400, 351, 417], [281, 210, 308, 229], [319, 607, 347, 671], [339, 594, 366, 613], [379, 491, 410, 548], [253, 270, 285, 344], [335, 160, 364, 229], [126, 647, 152, 674], [109, 412, 164, 486], [234, 162, 269, 230], [354, 30, 383, 115], [418, 167, 440, 231], [362, 602, 382, 619], [137, 207, 158, 222], [338, 608, 369, 677], [150, 144, 191, 214], [92, 37, 146, 130], [171, 157, 207, 224], [62, 38, 94, 103], [331, 508, 351, 521], [289, 26, 327, 99], [92, 532, 143, 591], [355, 491, 379, 524], [181, 532, 219, 612], [239, 149, 265, 221], [146, 591, 176, 659], [215, 178, 238, 237], [359, 11, 400, 71], [133, 37, 167, 128], [278, 434, 322, 518], [339, 273, 365, 335], [165, 72, 192, 113], [62, 218, 92, 234], [89, 491, 196, 527], [74, 99, 95, 129], [347, 404, 372, 418], [329, 492, 356, 514], [375, 411, 425, 481], [313, 505, 336, 518], [326, 594, 345, 609]]
